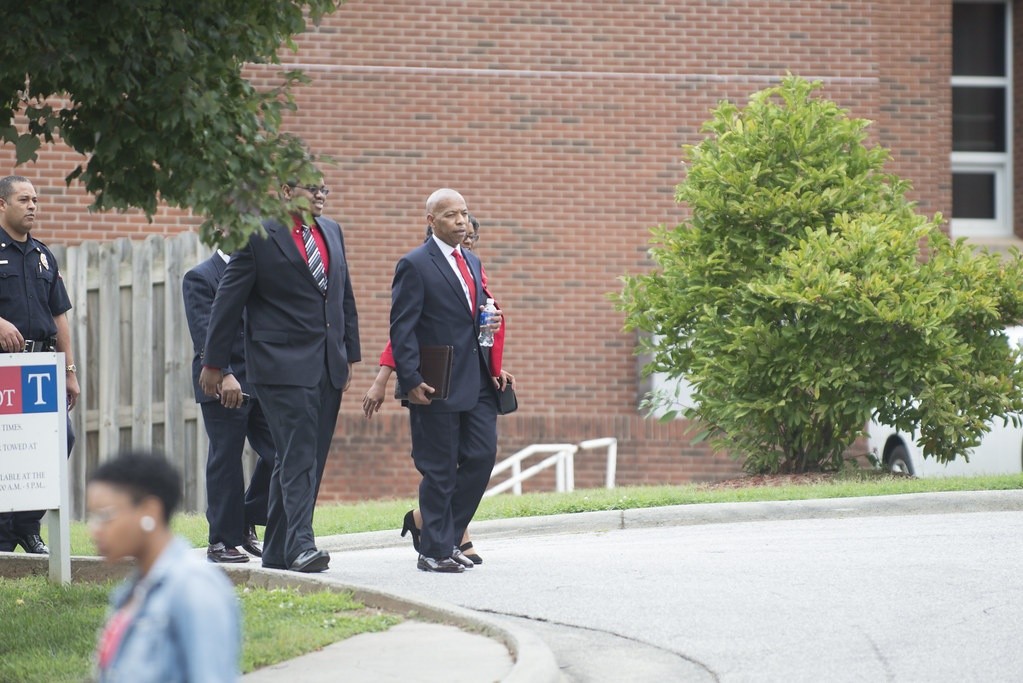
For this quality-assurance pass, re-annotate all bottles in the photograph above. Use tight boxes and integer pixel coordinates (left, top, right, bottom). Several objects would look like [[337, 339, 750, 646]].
[[478, 298, 496, 347]]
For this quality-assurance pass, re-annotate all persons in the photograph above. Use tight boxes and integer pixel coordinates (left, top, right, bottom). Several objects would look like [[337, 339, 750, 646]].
[[181, 170, 516, 573], [84, 451, 240, 683], [0, 175, 81, 555]]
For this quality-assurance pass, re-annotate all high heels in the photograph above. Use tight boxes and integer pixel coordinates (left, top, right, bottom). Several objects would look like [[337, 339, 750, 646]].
[[400, 509, 422, 554], [460, 542, 482, 564]]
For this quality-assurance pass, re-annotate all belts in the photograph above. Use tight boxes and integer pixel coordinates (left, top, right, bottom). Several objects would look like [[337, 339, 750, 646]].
[[22, 336, 57, 352]]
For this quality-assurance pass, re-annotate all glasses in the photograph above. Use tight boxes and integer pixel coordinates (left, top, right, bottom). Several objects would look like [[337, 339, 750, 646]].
[[290, 185, 329, 196], [465, 233, 479, 242]]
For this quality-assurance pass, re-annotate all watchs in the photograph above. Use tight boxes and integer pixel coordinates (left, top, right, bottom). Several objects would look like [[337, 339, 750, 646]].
[[66, 364, 76, 373]]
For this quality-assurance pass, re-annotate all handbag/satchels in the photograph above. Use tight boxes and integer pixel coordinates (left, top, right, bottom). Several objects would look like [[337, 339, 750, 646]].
[[498, 378, 518, 415]]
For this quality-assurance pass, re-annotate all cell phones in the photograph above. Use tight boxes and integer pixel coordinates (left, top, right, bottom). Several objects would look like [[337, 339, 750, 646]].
[[218, 393, 250, 409]]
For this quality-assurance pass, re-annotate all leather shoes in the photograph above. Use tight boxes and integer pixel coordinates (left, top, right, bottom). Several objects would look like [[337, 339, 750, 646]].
[[261, 560, 329, 572], [287, 548, 330, 574], [452, 544, 474, 568], [207, 541, 250, 563], [416, 553, 465, 573], [241, 517, 264, 557]]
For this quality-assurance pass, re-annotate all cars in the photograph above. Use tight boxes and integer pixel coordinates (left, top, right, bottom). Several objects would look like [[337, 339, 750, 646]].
[[868, 326, 1023, 476]]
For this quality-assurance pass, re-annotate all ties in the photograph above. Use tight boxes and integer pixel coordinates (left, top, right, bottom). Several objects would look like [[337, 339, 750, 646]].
[[302, 219, 328, 294], [451, 249, 477, 320]]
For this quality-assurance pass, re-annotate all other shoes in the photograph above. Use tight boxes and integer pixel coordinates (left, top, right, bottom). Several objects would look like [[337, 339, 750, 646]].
[[5, 517, 49, 555]]
